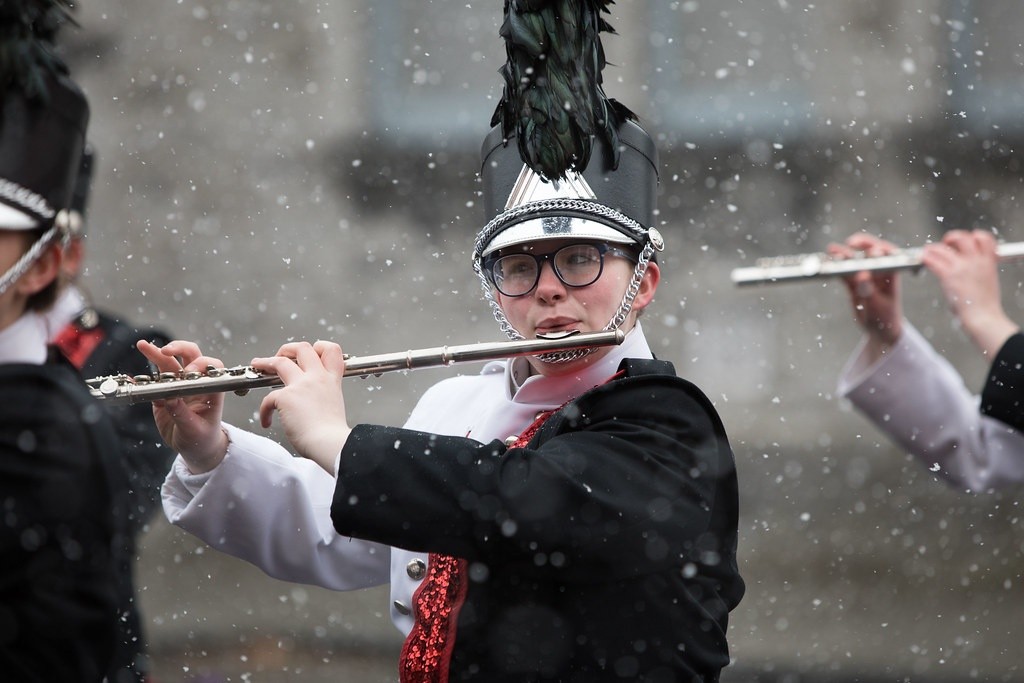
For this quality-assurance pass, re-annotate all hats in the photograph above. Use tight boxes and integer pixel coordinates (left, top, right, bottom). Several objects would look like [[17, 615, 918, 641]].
[[65, 153, 97, 233], [472, 0, 668, 259], [0, 0, 90, 230]]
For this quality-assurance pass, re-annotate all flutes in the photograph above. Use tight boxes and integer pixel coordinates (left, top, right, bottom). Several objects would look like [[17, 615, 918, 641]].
[[729, 239, 1024, 289], [82, 328, 625, 407]]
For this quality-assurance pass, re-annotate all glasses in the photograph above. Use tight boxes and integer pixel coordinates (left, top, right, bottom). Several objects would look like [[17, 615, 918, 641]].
[[481, 241, 641, 297]]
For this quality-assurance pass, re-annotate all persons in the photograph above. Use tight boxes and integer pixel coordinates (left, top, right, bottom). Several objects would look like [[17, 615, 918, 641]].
[[822, 229, 1024, 503], [1, 44, 182, 682], [135, 105, 744, 683]]
